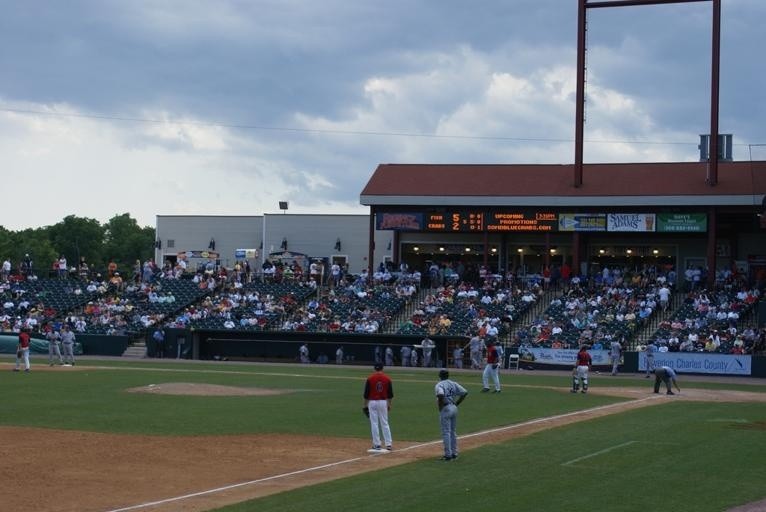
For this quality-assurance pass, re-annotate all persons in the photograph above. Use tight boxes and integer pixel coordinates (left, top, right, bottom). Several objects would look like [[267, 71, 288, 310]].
[[435, 368, 468, 461], [1, 256, 766, 394], [361, 361, 394, 452]]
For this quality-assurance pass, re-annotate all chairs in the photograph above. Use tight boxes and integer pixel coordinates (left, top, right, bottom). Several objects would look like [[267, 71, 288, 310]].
[[0, 272, 766, 353]]
